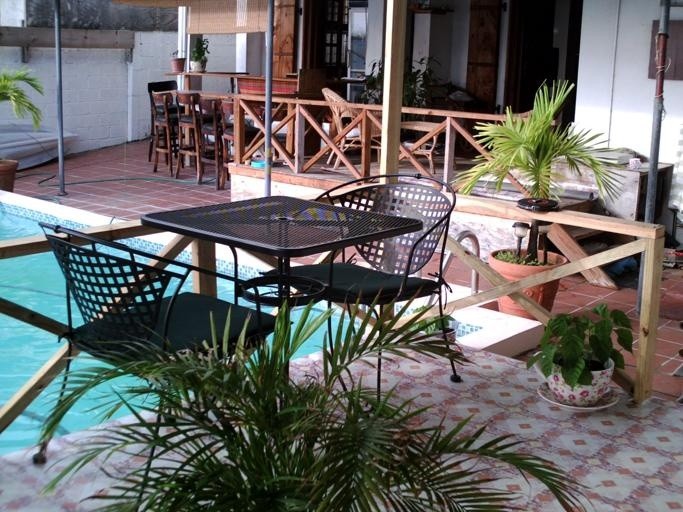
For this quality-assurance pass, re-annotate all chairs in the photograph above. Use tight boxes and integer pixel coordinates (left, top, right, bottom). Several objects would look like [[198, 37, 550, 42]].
[[148, 81, 383, 189], [425, 81, 500, 154], [264, 173, 460, 403], [34, 221, 274, 512]]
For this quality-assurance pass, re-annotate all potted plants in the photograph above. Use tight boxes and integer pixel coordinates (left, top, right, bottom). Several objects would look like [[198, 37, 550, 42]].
[[0, 64, 45, 192], [445, 77, 627, 317], [189, 37, 210, 73], [170, 50, 186, 73]]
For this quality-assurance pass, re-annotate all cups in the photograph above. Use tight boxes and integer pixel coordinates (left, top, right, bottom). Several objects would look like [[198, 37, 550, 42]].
[[629, 157, 641, 171]]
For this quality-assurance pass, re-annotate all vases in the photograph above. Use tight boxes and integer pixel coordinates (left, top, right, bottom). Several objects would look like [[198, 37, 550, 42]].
[[526, 304, 634, 407]]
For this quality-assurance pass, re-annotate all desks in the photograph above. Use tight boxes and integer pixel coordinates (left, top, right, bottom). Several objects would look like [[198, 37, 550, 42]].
[[396, 121, 446, 174], [141, 194, 423, 411]]
